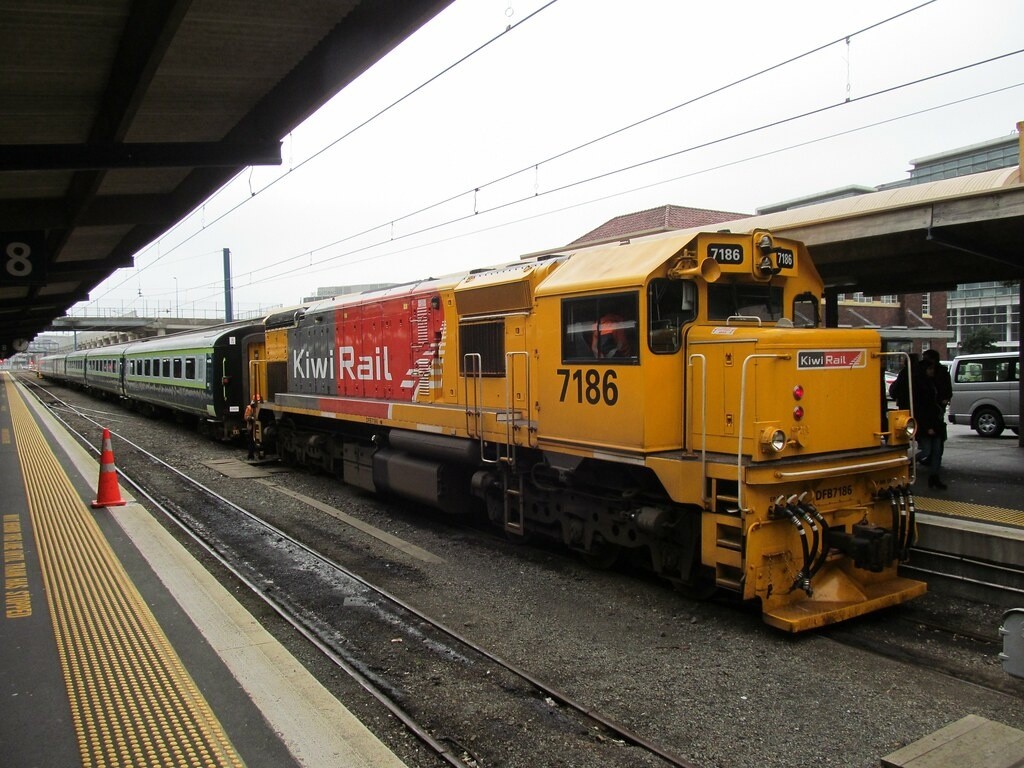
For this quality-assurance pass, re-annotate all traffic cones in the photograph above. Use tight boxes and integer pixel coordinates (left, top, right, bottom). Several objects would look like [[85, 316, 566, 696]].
[[91, 428, 126, 507]]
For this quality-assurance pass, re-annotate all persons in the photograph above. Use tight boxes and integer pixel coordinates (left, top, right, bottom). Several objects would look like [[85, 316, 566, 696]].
[[889, 349, 953, 487], [591, 314, 631, 358]]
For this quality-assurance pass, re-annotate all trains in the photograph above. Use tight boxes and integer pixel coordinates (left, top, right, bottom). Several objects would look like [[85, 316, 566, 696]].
[[37, 227, 927, 632]]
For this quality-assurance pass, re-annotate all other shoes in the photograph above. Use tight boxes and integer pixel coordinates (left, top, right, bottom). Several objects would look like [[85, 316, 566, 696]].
[[919, 458, 928, 466]]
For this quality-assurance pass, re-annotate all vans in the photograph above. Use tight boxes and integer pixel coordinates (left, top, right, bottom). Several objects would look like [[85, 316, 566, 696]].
[[947, 352, 1020, 436]]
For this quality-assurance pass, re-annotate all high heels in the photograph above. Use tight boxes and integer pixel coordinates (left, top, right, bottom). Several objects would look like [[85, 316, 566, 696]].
[[928, 474, 947, 490]]
